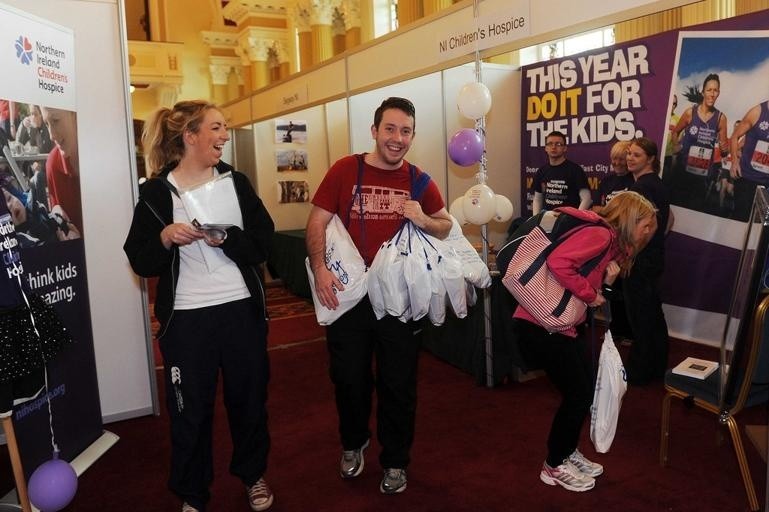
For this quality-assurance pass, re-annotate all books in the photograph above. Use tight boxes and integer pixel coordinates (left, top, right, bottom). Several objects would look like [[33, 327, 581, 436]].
[[672, 356, 719, 381], [191, 220, 233, 245]]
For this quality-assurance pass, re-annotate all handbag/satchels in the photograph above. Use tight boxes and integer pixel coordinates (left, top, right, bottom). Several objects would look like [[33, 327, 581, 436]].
[[588, 328, 630, 454], [305, 154, 492, 327], [500, 223, 614, 337]]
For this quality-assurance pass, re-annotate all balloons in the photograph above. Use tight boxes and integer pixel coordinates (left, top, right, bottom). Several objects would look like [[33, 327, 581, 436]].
[[447, 128, 483, 166], [28, 451, 78, 512], [456, 82, 492, 120], [449, 184, 514, 228]]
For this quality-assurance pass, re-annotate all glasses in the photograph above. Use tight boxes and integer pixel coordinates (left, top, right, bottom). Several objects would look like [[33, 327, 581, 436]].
[[544, 141, 564, 147]]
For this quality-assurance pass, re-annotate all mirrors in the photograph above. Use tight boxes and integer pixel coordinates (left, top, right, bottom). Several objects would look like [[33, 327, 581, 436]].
[[718, 184, 769, 408]]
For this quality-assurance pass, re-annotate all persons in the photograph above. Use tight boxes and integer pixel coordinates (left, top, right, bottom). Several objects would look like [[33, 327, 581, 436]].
[[287, 121, 294, 135], [123, 100, 276, 511], [1, 99, 83, 241], [669, 73, 769, 222], [287, 152, 305, 170], [530, 131, 592, 213], [595, 138, 672, 385], [512, 191, 654, 494], [305, 97, 451, 496]]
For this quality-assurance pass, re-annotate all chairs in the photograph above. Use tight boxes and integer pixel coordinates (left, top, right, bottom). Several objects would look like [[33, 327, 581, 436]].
[[658, 295, 769, 511]]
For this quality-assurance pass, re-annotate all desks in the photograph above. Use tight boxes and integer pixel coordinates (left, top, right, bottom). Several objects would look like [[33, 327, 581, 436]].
[[420, 269, 546, 385], [272, 228, 313, 304]]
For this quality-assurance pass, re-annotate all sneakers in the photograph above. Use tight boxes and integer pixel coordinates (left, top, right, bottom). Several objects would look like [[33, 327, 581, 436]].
[[539, 459, 596, 492], [246, 475, 273, 511], [568, 448, 604, 478], [339, 439, 369, 479], [183, 502, 198, 512], [379, 467, 407, 494]]
